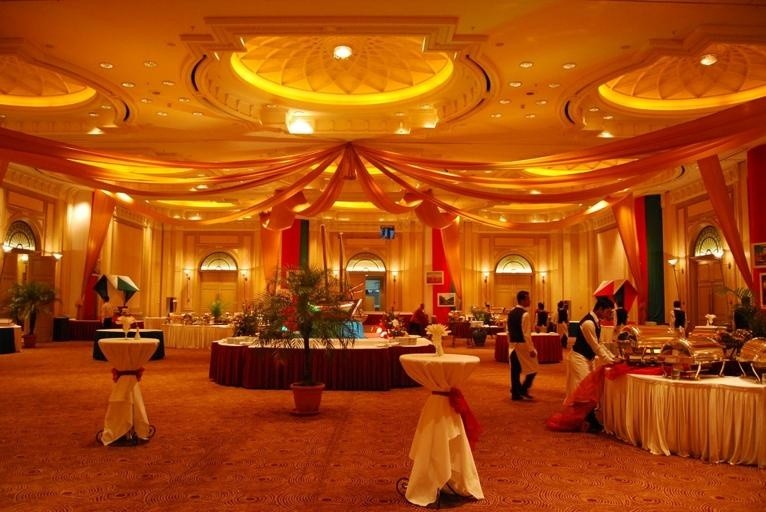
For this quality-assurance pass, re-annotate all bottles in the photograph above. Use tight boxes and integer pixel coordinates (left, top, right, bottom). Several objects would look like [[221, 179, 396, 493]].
[[134, 327, 141, 340]]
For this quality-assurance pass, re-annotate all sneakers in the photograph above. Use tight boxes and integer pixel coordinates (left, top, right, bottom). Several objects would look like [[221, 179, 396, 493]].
[[512, 391, 533, 400], [591, 420, 604, 430]]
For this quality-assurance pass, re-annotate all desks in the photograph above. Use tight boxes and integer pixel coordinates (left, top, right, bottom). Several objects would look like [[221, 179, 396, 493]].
[[135, 313, 219, 350], [397, 353, 481, 510], [210, 334, 434, 390], [96, 336, 159, 447], [92, 328, 166, 363]]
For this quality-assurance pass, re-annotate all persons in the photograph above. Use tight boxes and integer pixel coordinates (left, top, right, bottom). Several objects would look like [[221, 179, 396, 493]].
[[556, 301, 570, 348], [732, 295, 754, 332], [533, 302, 549, 326], [612, 300, 630, 326], [566, 295, 622, 433], [410, 303, 428, 321], [101, 295, 117, 327], [505, 290, 540, 400], [668, 300, 688, 338]]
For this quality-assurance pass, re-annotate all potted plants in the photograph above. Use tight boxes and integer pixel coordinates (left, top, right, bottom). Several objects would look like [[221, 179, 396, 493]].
[[1, 279, 63, 347], [246, 264, 368, 415]]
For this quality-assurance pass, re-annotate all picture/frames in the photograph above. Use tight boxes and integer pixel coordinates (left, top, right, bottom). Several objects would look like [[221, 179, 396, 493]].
[[424, 271, 456, 307], [750, 241, 765, 309]]
